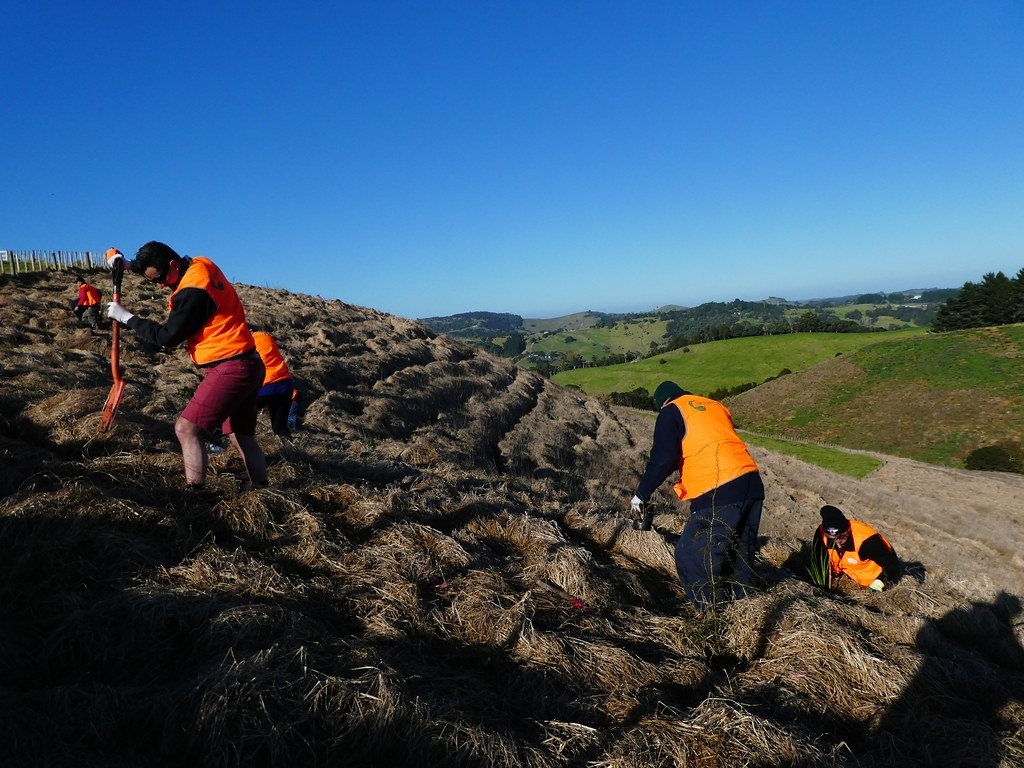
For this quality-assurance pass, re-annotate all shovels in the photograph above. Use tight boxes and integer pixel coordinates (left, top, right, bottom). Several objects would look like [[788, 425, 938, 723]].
[[95, 256, 126, 434]]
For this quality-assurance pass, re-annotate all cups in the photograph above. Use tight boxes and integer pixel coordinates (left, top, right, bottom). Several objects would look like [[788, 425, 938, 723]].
[[632, 504, 654, 530]]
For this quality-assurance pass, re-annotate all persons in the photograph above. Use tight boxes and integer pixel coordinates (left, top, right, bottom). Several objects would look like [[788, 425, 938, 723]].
[[813, 505, 899, 594], [631, 381, 766, 618], [287, 387, 299, 432], [72, 276, 104, 332], [247, 323, 293, 448], [105, 241, 270, 499]]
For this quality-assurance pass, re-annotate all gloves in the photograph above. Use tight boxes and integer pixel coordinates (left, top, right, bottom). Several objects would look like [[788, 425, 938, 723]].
[[108, 254, 131, 271], [106, 302, 134, 325], [630, 495, 644, 515], [869, 578, 885, 592]]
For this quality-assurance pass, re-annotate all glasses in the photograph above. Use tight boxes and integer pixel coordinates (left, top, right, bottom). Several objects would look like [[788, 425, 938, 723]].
[[147, 266, 168, 284]]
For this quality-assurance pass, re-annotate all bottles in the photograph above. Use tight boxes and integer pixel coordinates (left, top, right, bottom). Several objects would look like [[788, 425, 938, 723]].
[[203, 441, 225, 454]]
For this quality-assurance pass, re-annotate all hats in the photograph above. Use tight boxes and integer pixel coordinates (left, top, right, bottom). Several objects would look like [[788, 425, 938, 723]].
[[819, 505, 851, 536], [654, 381, 684, 407]]
